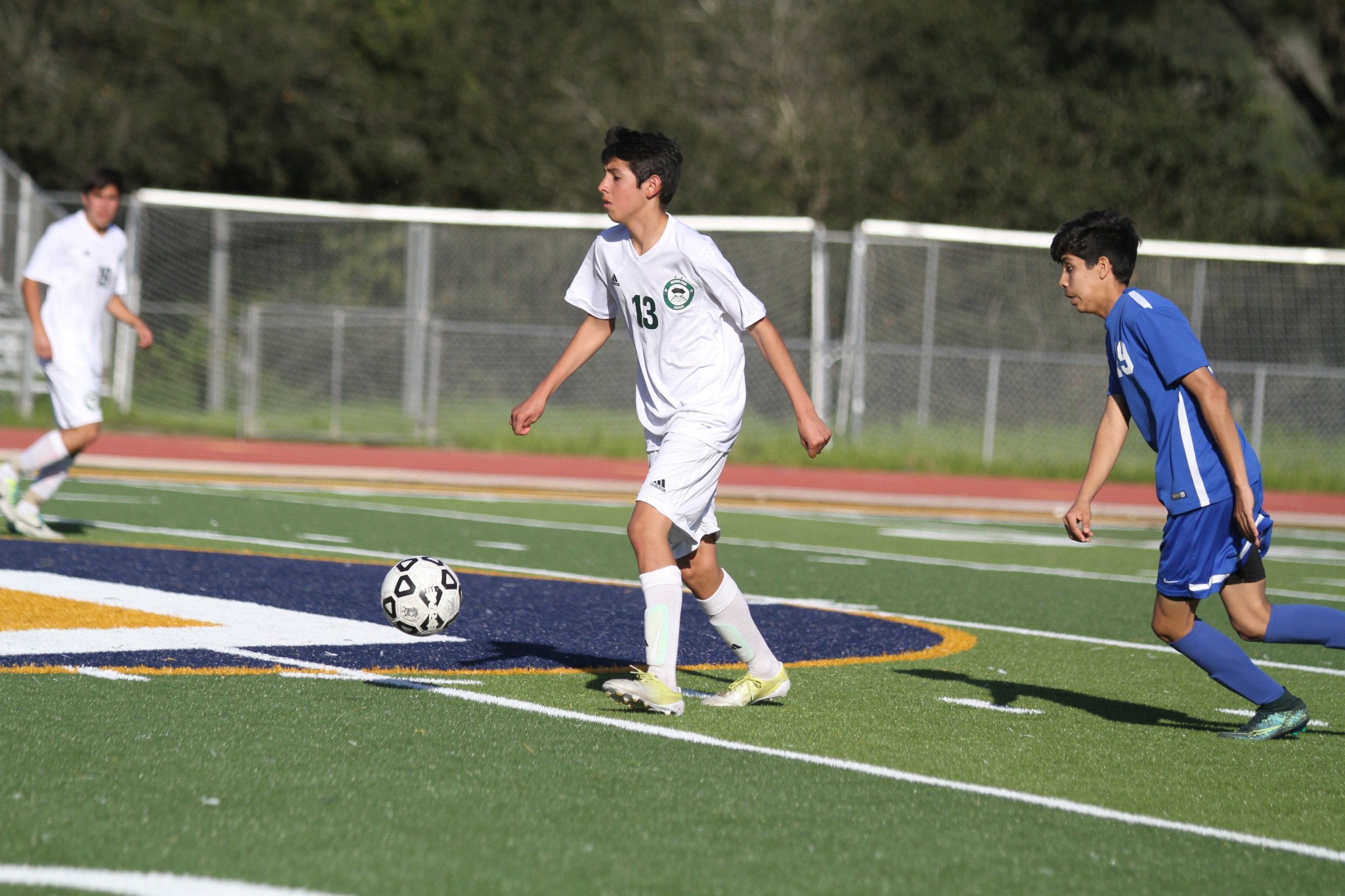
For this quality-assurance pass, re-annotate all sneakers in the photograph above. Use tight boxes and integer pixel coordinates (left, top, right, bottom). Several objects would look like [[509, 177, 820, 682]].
[[1218, 700, 1311, 740], [602, 665, 685, 718], [0, 462, 19, 523], [700, 660, 791, 706], [9, 506, 65, 541]]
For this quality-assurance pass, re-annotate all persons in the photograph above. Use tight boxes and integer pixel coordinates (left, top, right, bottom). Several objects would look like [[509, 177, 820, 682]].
[[1050, 211, 1345, 739], [0, 169, 154, 540], [509, 126, 832, 713]]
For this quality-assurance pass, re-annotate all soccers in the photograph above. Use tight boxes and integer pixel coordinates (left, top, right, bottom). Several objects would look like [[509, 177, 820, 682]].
[[380, 555, 461, 636]]
